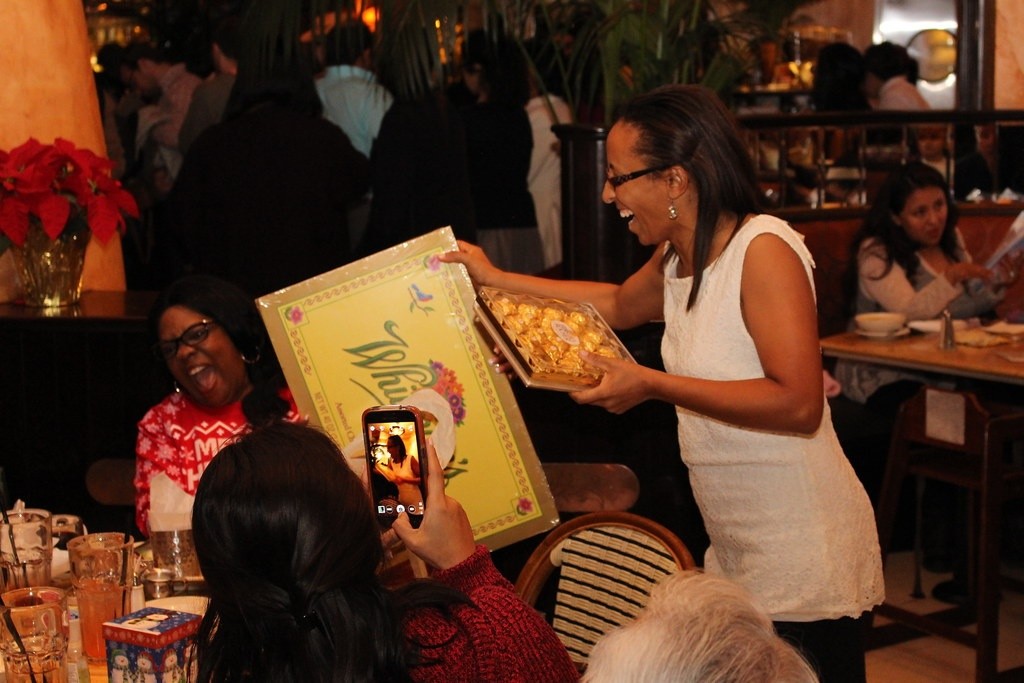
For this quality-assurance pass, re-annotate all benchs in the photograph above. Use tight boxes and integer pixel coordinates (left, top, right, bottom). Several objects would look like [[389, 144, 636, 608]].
[[782, 207, 1023, 321]]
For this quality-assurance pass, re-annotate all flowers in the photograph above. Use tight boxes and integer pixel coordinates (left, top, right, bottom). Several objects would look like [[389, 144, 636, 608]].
[[0, 138, 139, 244]]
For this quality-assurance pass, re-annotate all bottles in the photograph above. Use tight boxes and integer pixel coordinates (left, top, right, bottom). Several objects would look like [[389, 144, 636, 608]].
[[130, 572, 145, 613], [67, 648, 91, 683]]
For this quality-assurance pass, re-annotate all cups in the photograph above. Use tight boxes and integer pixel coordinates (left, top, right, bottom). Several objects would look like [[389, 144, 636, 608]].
[[0, 587, 70, 683], [0, 509, 52, 594], [67, 532, 134, 663], [143, 568, 188, 600]]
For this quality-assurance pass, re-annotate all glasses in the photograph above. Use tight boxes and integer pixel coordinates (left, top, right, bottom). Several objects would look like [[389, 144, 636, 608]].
[[153, 318, 218, 361], [603, 160, 685, 192], [388, 446, 394, 449]]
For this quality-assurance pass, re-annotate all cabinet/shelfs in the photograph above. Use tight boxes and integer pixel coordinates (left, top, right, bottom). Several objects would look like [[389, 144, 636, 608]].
[[0, 289, 173, 543]]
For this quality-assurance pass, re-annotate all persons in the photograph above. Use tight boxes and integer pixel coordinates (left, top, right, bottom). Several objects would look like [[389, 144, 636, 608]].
[[134, 274, 518, 539], [191, 388, 578, 683], [582, 569, 819, 682], [93, 15, 585, 331], [373, 435, 424, 516], [835, 158, 1007, 566], [441, 83, 887, 682], [812, 41, 930, 111]]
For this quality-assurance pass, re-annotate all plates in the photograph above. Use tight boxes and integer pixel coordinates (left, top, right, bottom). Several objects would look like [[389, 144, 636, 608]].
[[855, 329, 911, 339], [908, 320, 968, 333]]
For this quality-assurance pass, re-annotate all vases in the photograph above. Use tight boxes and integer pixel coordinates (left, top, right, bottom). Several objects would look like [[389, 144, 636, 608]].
[[9, 228, 88, 305]]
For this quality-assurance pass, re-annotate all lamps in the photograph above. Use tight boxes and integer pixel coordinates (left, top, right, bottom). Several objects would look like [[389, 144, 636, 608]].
[[370, 424, 414, 437]]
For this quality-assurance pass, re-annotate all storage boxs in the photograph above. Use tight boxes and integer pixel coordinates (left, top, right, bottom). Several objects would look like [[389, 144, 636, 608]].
[[102, 607, 202, 683]]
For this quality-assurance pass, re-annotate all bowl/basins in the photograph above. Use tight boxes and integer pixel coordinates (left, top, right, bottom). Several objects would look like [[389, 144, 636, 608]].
[[855, 313, 906, 333]]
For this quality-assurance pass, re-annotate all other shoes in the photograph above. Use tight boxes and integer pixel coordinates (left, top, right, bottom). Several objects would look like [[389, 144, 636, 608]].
[[928, 568, 969, 603]]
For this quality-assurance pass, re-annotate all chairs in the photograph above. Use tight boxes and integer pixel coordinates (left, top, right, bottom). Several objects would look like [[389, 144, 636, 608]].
[[518, 462, 693, 675]]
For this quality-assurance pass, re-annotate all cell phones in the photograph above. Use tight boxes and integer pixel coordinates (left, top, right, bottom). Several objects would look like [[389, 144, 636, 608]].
[[362, 404, 429, 528]]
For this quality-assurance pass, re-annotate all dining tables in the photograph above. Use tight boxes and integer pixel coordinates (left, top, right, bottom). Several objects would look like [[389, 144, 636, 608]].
[[820, 315, 1024, 683]]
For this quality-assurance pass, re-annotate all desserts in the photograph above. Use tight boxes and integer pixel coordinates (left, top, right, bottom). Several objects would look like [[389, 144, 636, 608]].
[[488, 298, 619, 376]]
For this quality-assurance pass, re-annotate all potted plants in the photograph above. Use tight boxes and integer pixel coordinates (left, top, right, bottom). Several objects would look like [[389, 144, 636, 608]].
[[549, 0, 730, 284]]
